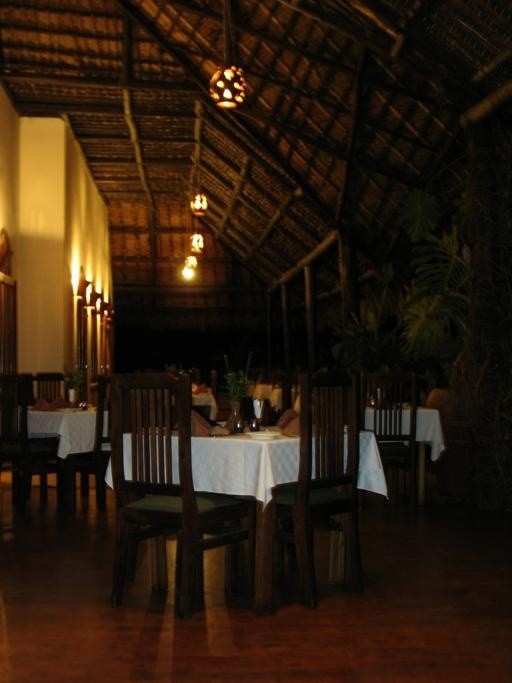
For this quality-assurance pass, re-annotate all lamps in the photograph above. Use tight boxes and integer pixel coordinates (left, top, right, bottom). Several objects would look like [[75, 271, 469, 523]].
[[191, 113, 207, 218], [208, 3, 246, 111]]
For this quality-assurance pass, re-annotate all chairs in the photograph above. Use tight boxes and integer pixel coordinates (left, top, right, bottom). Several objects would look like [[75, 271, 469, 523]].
[[113, 371, 419, 611], [4, 371, 136, 522]]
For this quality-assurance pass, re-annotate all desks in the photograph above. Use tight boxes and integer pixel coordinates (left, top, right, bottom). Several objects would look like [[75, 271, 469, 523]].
[[367, 403, 442, 504]]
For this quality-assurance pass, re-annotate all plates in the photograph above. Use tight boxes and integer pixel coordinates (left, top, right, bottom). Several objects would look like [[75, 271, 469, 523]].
[[245, 431, 281, 440], [55, 408, 83, 413]]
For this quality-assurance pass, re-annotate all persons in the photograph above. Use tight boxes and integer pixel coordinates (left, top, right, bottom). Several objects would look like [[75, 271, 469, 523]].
[[427, 368, 474, 427], [191, 366, 218, 422], [238, 377, 277, 427]]
